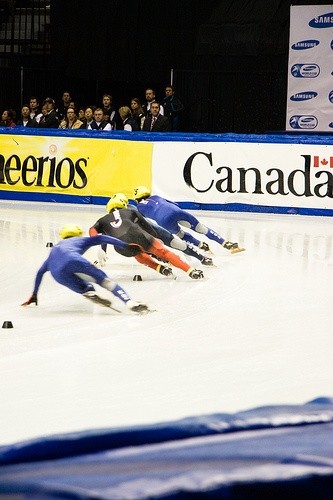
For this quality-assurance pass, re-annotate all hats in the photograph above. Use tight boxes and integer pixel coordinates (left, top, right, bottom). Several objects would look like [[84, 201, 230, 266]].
[[45, 98, 55, 106]]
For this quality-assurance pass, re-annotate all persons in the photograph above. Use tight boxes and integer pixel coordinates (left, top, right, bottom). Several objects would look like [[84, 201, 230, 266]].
[[20, 225, 149, 314], [93, 192, 217, 268], [134, 187, 245, 256], [89, 199, 205, 279], [0, 86, 184, 132]]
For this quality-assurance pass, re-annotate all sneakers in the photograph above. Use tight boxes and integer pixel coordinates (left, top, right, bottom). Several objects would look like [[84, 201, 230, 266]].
[[189, 270, 205, 281], [201, 258, 217, 268], [159, 266, 177, 281], [84, 291, 122, 313], [127, 299, 151, 316], [223, 241, 246, 255], [200, 242, 212, 254]]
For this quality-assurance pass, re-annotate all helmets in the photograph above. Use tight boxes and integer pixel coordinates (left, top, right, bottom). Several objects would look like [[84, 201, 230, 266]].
[[106, 198, 126, 213], [111, 193, 129, 202], [133, 186, 150, 200], [60, 225, 84, 239]]
